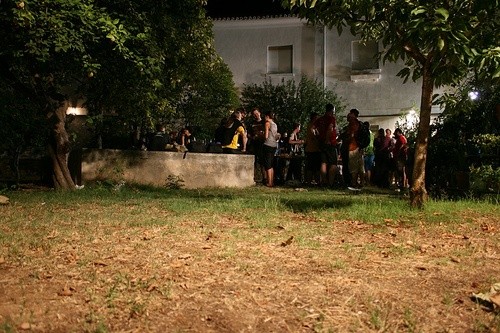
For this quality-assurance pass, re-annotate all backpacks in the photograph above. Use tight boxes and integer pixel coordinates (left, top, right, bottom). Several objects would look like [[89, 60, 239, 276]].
[[354, 120, 370, 148]]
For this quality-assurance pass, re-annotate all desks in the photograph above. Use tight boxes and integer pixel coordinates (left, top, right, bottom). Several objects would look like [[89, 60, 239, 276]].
[[274, 154, 306, 184]]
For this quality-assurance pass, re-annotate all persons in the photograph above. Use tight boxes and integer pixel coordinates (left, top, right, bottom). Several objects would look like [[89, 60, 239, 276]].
[[306, 103, 340, 191], [341, 108, 368, 191], [150, 124, 196, 152], [222, 109, 281, 186], [365, 128, 415, 190], [282, 121, 305, 191]]
